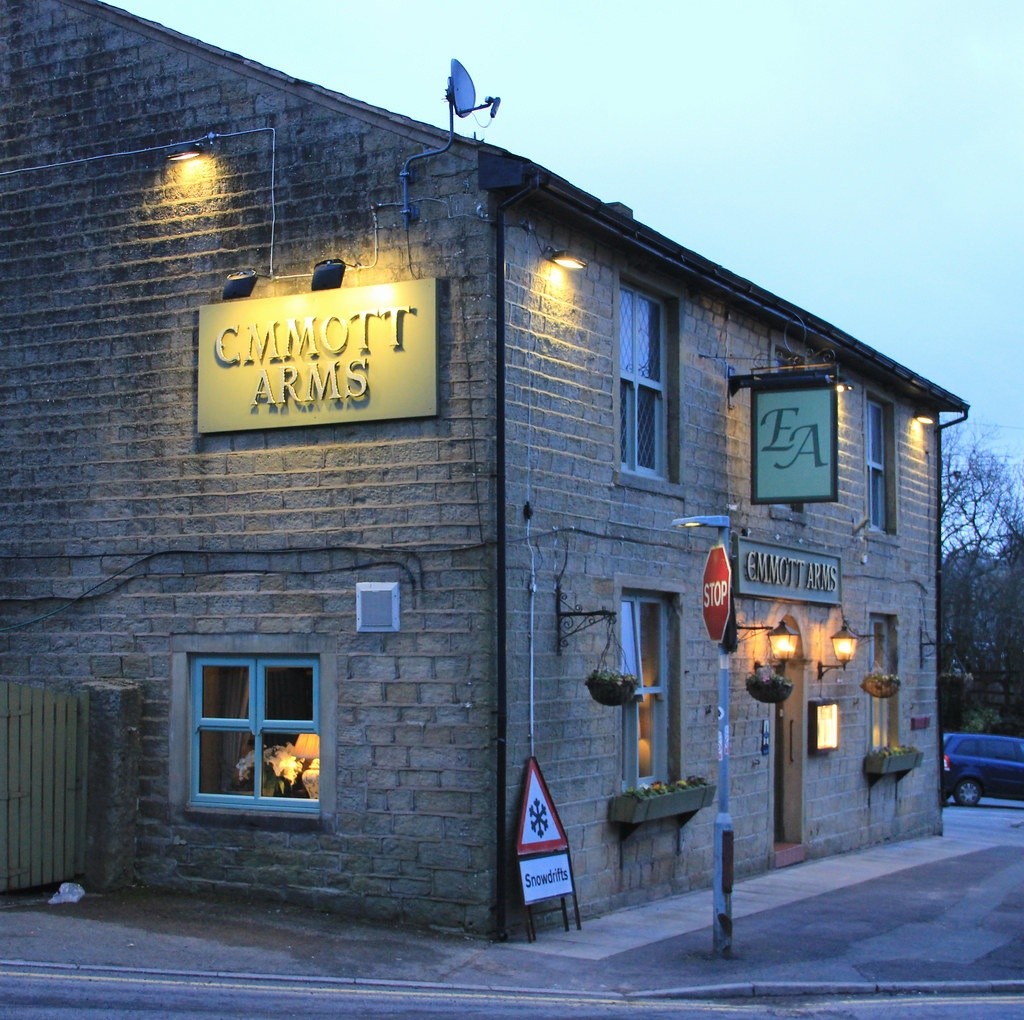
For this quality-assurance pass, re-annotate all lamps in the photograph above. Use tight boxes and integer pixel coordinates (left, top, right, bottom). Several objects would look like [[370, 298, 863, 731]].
[[222, 272, 259, 301], [312, 260, 348, 292], [818, 622, 859, 681], [290, 733, 320, 769], [551, 249, 588, 270], [167, 144, 202, 161], [752, 620, 799, 672]]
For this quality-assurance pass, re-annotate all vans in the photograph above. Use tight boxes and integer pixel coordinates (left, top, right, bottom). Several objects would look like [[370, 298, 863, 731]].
[[941, 734, 1024, 807]]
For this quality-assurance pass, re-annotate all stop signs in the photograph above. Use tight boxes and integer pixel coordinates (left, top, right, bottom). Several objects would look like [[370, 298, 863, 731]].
[[700, 543, 730, 645]]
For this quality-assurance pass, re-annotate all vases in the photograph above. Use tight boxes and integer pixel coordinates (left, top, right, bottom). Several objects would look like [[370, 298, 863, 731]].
[[746, 682, 793, 703], [864, 682, 900, 698], [585, 680, 637, 705], [864, 751, 923, 773], [611, 785, 717, 824]]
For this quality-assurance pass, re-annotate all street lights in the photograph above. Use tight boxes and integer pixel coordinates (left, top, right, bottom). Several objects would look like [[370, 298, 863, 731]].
[[670, 515, 739, 959]]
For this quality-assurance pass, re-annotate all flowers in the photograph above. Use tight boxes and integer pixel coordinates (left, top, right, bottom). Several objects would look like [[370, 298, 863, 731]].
[[860, 672, 902, 687], [868, 744, 920, 758], [622, 775, 714, 803], [744, 665, 794, 688], [585, 666, 637, 686]]
